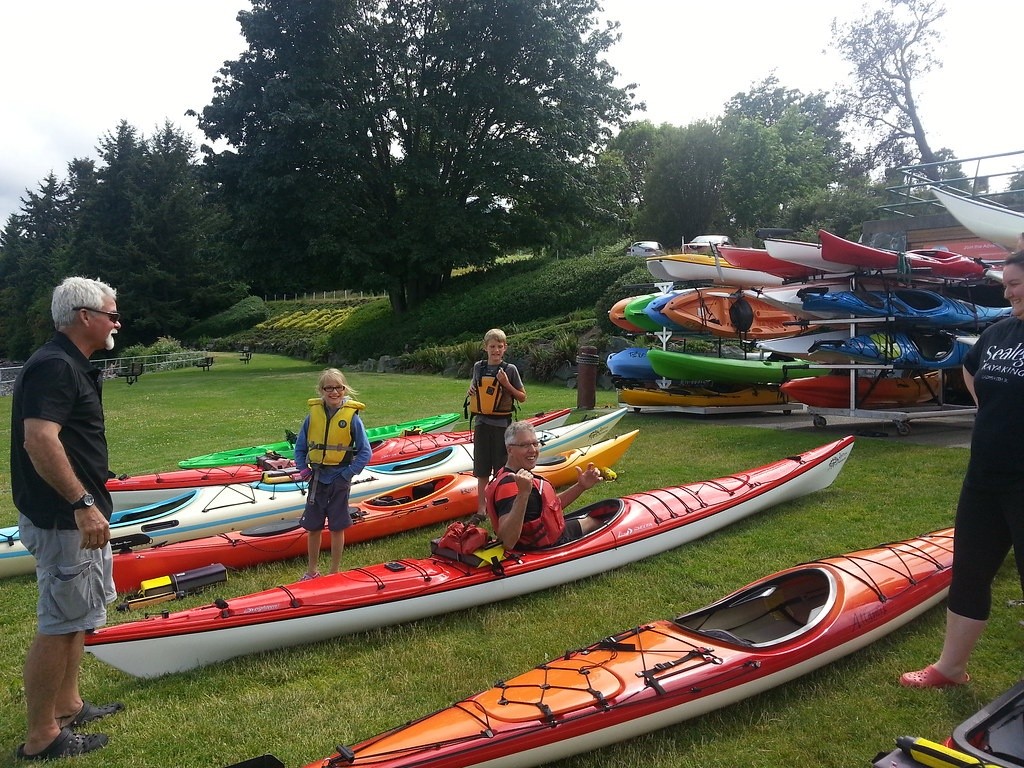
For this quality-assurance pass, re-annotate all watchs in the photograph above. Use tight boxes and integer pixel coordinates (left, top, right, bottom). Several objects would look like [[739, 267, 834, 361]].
[[71, 493, 95, 510]]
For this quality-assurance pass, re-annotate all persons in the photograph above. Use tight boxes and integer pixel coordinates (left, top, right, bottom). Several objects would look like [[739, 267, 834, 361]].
[[294, 368, 372, 582], [485, 421, 610, 550], [466, 329, 526, 529], [10, 276, 125, 760], [901, 251, 1024, 688]]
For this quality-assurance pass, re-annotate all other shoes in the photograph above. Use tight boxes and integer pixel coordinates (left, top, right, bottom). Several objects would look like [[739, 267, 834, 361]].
[[299, 572, 319, 581]]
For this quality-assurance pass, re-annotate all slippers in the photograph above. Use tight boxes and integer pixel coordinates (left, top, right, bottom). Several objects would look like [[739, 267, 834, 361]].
[[16, 726, 108, 763], [464, 513, 486, 526], [59, 699, 125, 731], [900, 666, 970, 689]]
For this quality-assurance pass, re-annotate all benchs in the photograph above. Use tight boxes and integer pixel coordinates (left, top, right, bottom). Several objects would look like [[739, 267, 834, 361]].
[[196, 357, 214, 372], [239, 352, 252, 365], [116, 363, 144, 385], [202, 344, 214, 352], [238, 346, 249, 355]]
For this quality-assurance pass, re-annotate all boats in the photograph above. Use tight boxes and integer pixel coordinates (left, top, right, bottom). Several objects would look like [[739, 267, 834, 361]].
[[107, 426, 643, 594], [80, 433, 858, 682], [178, 411, 462, 471], [0, 404, 631, 580], [291, 525, 968, 768], [601, 185, 1024, 406], [103, 405, 571, 513]]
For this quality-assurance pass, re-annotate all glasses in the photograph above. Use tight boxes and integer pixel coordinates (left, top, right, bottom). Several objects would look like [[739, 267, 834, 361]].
[[322, 385, 345, 393], [508, 441, 538, 447], [72, 307, 120, 324]]
